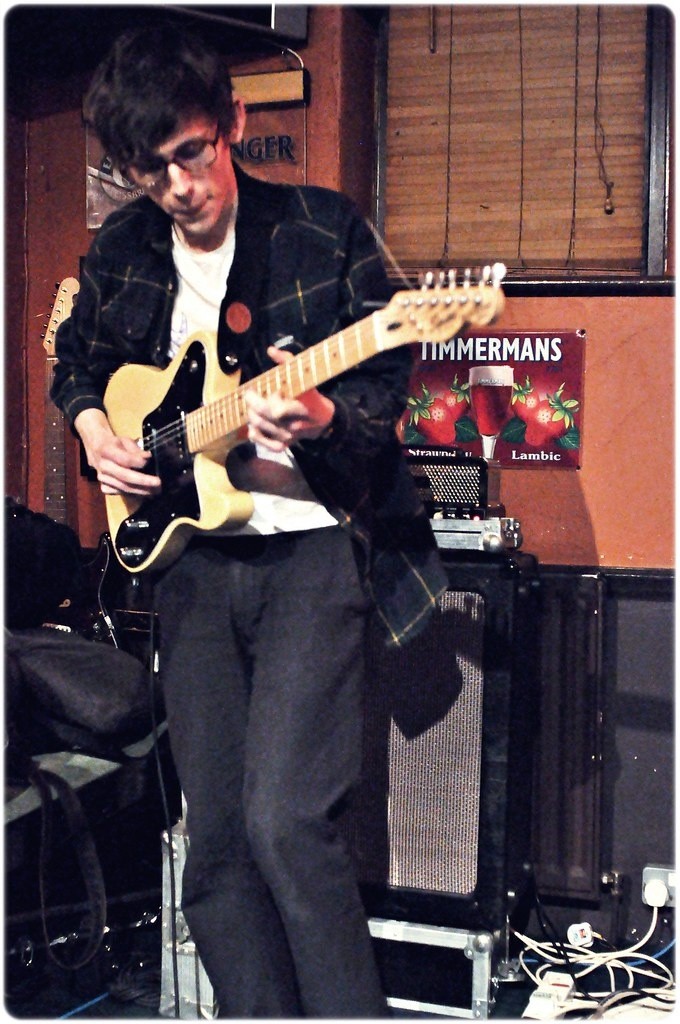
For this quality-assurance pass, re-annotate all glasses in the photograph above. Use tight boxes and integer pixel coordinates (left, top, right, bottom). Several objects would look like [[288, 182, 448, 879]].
[[122, 99, 226, 189]]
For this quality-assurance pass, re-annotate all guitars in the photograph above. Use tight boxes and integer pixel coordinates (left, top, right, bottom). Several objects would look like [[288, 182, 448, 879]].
[[100, 259, 508, 576], [39, 275, 82, 528]]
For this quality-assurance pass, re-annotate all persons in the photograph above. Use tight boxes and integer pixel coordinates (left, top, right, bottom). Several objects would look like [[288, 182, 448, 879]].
[[48, 19, 464, 1020]]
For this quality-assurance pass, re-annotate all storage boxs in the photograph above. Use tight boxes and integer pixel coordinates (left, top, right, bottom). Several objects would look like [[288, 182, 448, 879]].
[[157, 824, 223, 1019], [362, 919, 509, 1021]]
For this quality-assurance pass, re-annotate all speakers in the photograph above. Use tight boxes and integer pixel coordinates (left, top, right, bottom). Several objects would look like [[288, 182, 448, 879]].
[[375, 553, 541, 937]]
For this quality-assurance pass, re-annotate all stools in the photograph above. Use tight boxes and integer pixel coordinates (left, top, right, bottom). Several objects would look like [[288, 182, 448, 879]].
[[4, 723, 169, 1018]]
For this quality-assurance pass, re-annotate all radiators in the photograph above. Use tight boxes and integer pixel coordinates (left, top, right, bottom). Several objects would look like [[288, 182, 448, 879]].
[[514, 560, 625, 947]]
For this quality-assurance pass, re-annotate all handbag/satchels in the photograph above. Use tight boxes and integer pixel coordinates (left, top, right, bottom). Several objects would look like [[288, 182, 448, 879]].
[[2, 620, 161, 983]]
